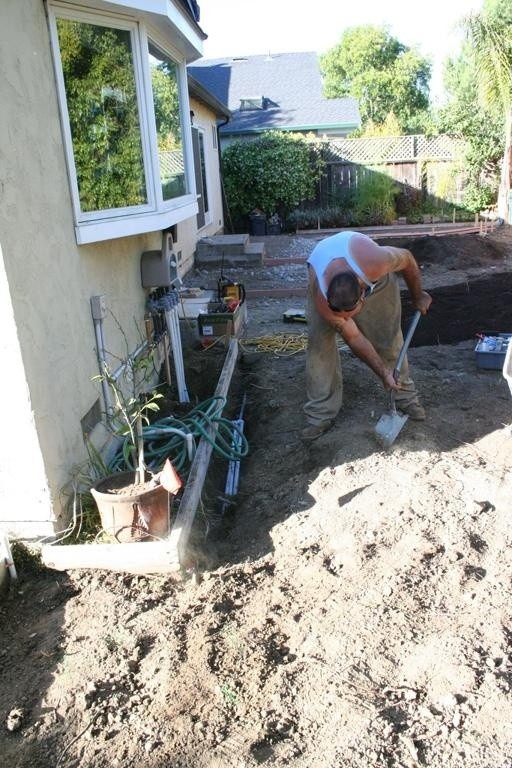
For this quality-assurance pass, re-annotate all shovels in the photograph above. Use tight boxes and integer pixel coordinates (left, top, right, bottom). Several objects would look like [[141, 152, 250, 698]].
[[373, 310, 424, 443]]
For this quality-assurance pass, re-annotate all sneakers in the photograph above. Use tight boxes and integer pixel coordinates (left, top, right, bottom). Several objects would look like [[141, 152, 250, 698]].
[[300, 414, 336, 446], [399, 401, 429, 423]]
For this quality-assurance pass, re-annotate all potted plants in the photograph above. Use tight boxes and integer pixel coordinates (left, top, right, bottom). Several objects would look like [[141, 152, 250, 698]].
[[88, 309, 179, 540]]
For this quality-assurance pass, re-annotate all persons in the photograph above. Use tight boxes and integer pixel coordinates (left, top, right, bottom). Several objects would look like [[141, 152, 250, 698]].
[[297, 229, 434, 443]]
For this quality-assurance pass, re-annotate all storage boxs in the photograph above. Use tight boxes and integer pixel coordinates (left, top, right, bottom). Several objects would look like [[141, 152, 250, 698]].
[[198, 302, 242, 335], [474, 332, 512, 370]]
